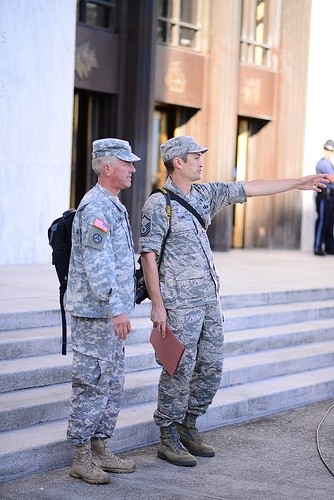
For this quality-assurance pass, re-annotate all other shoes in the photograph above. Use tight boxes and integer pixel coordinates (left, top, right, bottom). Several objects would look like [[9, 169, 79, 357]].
[[314, 250, 326, 256]]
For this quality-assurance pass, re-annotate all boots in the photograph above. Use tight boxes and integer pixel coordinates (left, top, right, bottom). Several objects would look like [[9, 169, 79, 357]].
[[69, 444, 111, 485], [157, 422, 197, 466], [177, 414, 215, 457], [91, 437, 136, 474]]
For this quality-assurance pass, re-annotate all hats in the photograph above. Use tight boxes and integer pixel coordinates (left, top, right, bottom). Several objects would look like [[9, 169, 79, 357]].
[[160, 135, 208, 163], [323, 140, 334, 151], [91, 138, 141, 163]]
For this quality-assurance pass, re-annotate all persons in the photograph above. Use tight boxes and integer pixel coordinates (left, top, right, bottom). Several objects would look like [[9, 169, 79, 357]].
[[138, 135, 329, 466], [314, 139, 334, 256], [63, 139, 140, 484]]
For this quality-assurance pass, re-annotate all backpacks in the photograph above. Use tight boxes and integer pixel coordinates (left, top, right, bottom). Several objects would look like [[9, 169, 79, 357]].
[[47, 208, 76, 290]]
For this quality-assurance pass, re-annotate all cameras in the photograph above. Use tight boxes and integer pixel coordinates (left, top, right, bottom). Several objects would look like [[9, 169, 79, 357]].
[[136, 280, 151, 304]]
